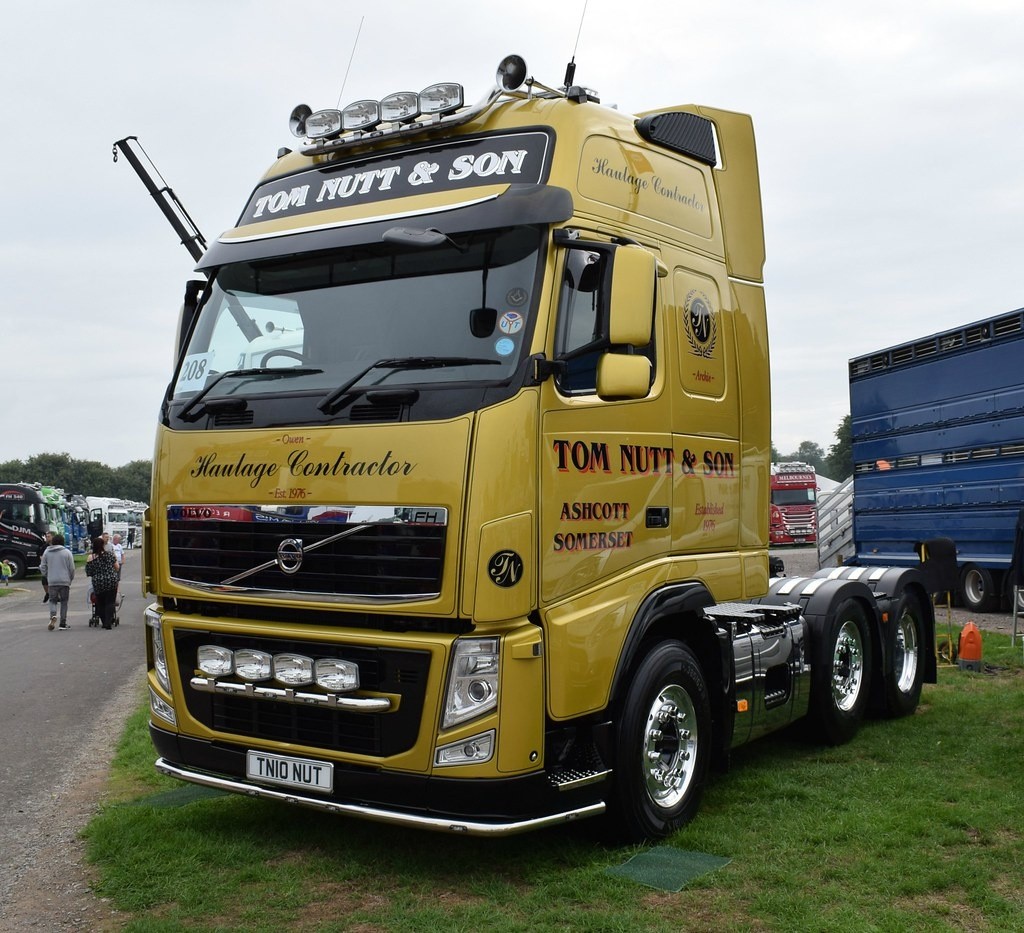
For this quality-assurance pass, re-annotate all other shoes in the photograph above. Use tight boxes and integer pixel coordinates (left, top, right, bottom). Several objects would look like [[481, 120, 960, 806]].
[[43, 594, 49, 603], [5, 582, 9, 586], [102, 625, 113, 630]]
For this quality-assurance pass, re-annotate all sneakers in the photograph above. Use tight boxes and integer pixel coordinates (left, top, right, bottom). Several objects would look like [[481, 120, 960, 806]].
[[47, 616, 57, 631], [59, 621, 71, 630]]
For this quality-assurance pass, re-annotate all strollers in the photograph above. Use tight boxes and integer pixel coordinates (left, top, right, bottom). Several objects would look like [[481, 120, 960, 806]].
[[87, 587, 125, 628]]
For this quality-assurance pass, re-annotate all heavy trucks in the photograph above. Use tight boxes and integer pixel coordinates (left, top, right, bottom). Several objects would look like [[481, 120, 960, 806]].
[[769, 461, 821, 548], [139, 55, 959, 840], [112, 137, 305, 367], [0, 482, 148, 578]]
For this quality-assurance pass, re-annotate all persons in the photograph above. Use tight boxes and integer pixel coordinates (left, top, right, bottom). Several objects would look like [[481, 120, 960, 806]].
[[127, 530, 134, 549], [39, 530, 60, 602], [39, 534, 75, 630], [97, 532, 126, 592], [0, 559, 11, 587], [85, 537, 119, 629]]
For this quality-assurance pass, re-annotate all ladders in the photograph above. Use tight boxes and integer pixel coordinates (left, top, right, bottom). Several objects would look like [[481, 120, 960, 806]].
[[1011, 584, 1024, 647]]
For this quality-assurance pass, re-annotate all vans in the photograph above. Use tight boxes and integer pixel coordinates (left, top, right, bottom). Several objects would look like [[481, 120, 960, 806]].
[[531, 461, 739, 632], [740, 454, 768, 590]]
